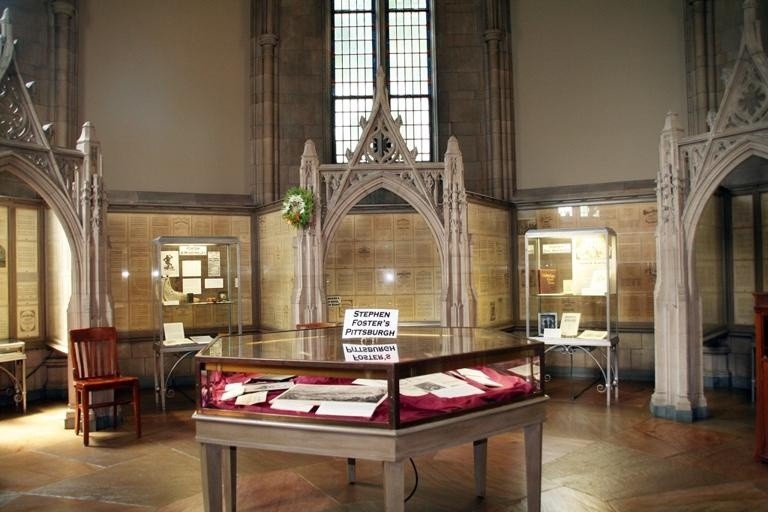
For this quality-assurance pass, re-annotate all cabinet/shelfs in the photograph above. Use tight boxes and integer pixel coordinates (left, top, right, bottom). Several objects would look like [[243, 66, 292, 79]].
[[153, 235, 242, 412], [0, 338, 27, 415], [524, 226, 619, 408], [192, 327, 549, 512]]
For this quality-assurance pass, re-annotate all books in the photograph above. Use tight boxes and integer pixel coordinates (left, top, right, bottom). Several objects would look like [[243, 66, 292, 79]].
[[537, 269, 557, 294]]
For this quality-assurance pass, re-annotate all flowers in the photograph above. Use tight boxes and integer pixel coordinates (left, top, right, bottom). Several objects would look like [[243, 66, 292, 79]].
[[281, 186, 314, 228]]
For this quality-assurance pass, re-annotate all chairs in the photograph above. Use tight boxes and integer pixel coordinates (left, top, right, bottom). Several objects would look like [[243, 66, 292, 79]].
[[70, 326, 141, 446]]
